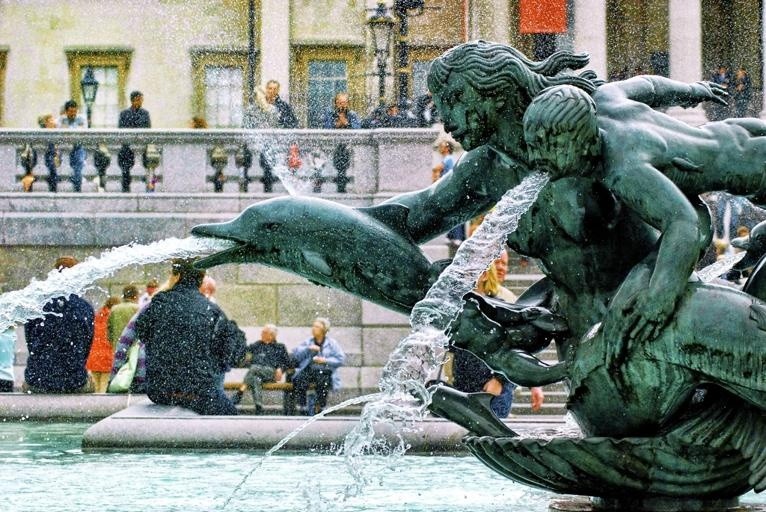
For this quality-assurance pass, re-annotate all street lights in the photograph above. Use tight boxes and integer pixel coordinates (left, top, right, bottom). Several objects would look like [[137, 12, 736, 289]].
[[77, 57, 99, 131], [363, 0, 397, 129]]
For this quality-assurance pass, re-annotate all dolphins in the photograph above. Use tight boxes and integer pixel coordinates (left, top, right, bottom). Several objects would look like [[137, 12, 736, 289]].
[[188, 193, 567, 387], [407, 381, 520, 438]]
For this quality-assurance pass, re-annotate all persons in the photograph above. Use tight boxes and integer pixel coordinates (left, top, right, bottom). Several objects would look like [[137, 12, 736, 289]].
[[290, 317, 344, 412], [37, 114, 55, 130], [450, 249, 545, 419], [433, 141, 468, 241], [22, 255, 245, 416], [1, 326, 18, 393], [524, 74, 766, 341], [230, 325, 290, 415], [712, 64, 751, 120], [117, 92, 151, 129], [328, 93, 440, 130], [245, 80, 299, 126], [53, 98, 88, 129], [385, 41, 716, 343]]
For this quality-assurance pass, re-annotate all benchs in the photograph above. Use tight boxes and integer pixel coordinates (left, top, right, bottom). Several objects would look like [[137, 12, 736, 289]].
[[221, 350, 332, 415]]
